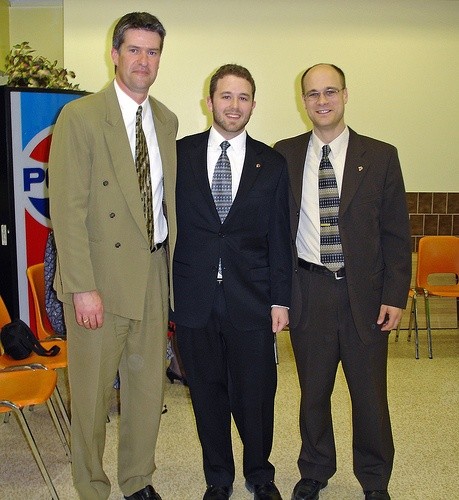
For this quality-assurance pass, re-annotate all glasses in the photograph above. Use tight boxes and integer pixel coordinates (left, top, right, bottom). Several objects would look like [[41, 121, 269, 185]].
[[304, 88, 344, 102]]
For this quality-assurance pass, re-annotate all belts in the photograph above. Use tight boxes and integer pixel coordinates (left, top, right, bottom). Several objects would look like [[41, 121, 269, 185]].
[[297, 256, 346, 281], [149, 243, 163, 254], [216, 279, 223, 288]]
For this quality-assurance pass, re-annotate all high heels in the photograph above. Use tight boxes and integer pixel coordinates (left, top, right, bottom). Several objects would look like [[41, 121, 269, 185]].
[[165, 367, 188, 387]]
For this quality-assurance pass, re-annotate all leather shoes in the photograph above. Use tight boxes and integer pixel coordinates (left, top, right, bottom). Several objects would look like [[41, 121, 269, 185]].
[[124, 485, 162, 500], [291, 478, 328, 500], [365, 489, 391, 500], [203, 483, 233, 500], [245, 479, 283, 500]]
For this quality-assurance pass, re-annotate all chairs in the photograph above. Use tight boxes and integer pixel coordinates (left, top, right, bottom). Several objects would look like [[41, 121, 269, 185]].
[[396, 234, 459, 360], [0, 263, 110, 500]]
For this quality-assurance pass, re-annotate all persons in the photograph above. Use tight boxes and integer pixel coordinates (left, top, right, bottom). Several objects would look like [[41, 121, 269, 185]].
[[44, 231, 189, 415], [171, 64, 292, 500], [49, 13, 179, 500], [272, 62, 413, 500]]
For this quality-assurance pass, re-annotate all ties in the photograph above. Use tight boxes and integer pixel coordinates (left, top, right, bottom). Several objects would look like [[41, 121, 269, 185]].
[[135, 105, 154, 252], [210, 140, 232, 276], [318, 145, 345, 273]]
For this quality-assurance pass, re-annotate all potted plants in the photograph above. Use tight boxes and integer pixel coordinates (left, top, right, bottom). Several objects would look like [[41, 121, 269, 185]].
[[0, 39, 82, 91]]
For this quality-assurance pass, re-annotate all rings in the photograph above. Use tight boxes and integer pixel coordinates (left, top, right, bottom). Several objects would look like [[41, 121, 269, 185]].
[[83, 318, 89, 323]]
